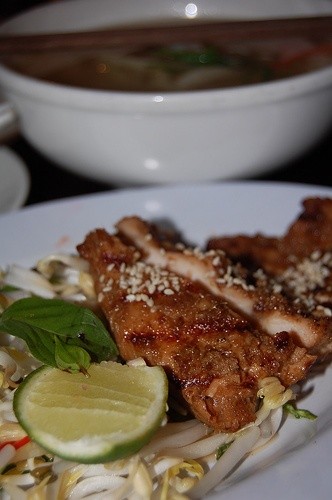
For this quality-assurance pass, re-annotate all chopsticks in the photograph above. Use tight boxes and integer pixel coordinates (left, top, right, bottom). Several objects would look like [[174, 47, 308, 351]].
[[0, 15, 332, 52]]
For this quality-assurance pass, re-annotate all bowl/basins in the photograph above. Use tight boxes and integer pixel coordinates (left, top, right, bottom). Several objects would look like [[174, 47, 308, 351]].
[[1, 1, 331, 185]]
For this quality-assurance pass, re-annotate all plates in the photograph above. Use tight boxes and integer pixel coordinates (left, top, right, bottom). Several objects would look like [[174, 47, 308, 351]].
[[1, 182, 332, 500], [1, 149, 29, 216]]
